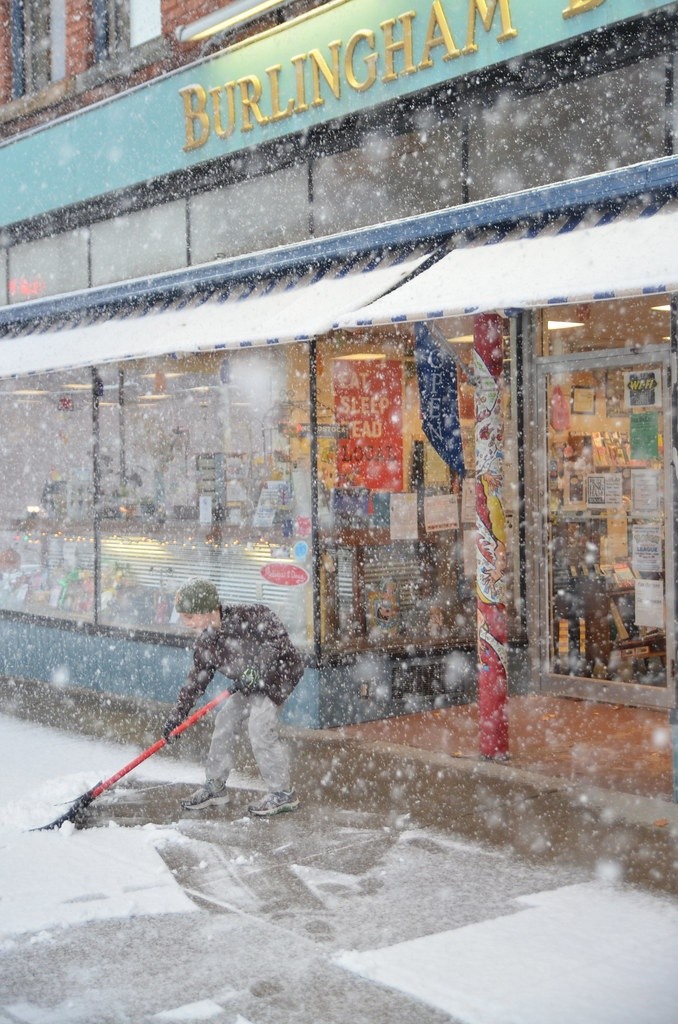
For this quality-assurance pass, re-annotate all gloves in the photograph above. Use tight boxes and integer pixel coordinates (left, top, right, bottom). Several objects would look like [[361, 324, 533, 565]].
[[239, 667, 260, 696], [162, 715, 181, 743]]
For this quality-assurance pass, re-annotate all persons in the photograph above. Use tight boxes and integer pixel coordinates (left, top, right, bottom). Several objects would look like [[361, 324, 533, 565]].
[[160, 577, 306, 817]]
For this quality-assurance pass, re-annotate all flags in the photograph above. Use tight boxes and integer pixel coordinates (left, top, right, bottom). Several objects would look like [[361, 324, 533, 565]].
[[412, 320, 469, 480]]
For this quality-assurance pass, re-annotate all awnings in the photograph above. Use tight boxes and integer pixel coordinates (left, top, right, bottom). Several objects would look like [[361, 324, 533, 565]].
[[0, 184, 677, 379]]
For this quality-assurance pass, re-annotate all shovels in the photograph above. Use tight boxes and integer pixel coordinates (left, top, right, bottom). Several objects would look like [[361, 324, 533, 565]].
[[18, 672, 258, 835]]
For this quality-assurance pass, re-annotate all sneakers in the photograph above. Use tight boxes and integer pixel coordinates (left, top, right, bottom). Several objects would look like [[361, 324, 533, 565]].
[[248, 786, 298, 817], [180, 781, 230, 810]]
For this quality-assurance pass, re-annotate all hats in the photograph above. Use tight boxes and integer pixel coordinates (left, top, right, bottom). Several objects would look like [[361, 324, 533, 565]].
[[175, 577, 221, 614]]
